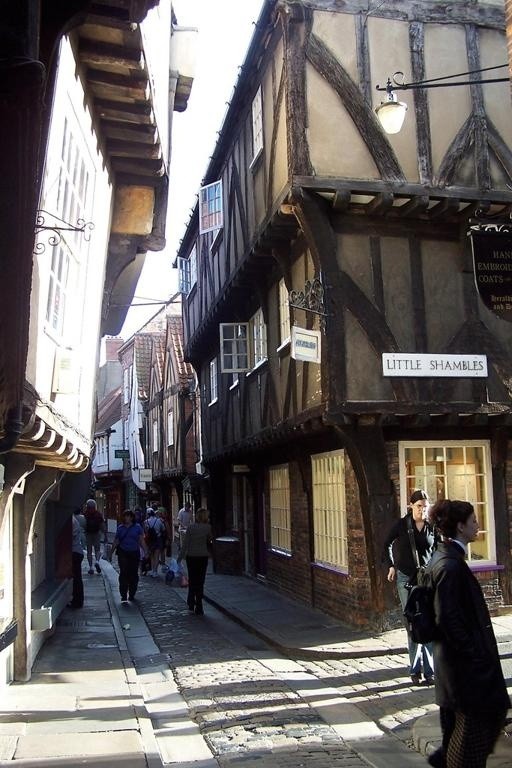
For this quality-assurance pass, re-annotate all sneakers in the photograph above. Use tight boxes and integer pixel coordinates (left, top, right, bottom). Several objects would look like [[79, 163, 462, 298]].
[[410, 675, 435, 686], [189, 604, 204, 616], [151, 572, 159, 578], [88, 563, 101, 574], [120, 593, 134, 601], [426, 749, 446, 768], [66, 601, 83, 609]]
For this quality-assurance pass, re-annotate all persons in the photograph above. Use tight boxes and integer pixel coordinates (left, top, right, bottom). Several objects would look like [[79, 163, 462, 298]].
[[110, 507, 150, 604], [83, 498, 109, 573], [132, 501, 174, 578], [418, 497, 512, 768], [67, 513, 85, 607], [177, 507, 216, 616], [383, 489, 446, 687], [74, 506, 88, 551], [177, 502, 194, 560]]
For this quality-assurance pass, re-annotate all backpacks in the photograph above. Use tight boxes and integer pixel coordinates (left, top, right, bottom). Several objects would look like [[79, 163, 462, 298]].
[[403, 552, 458, 643], [146, 518, 158, 541]]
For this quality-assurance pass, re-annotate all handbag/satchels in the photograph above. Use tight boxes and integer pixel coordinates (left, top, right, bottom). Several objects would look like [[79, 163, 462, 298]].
[[206, 526, 215, 553], [116, 543, 121, 555], [167, 539, 171, 556], [101, 543, 114, 562]]
